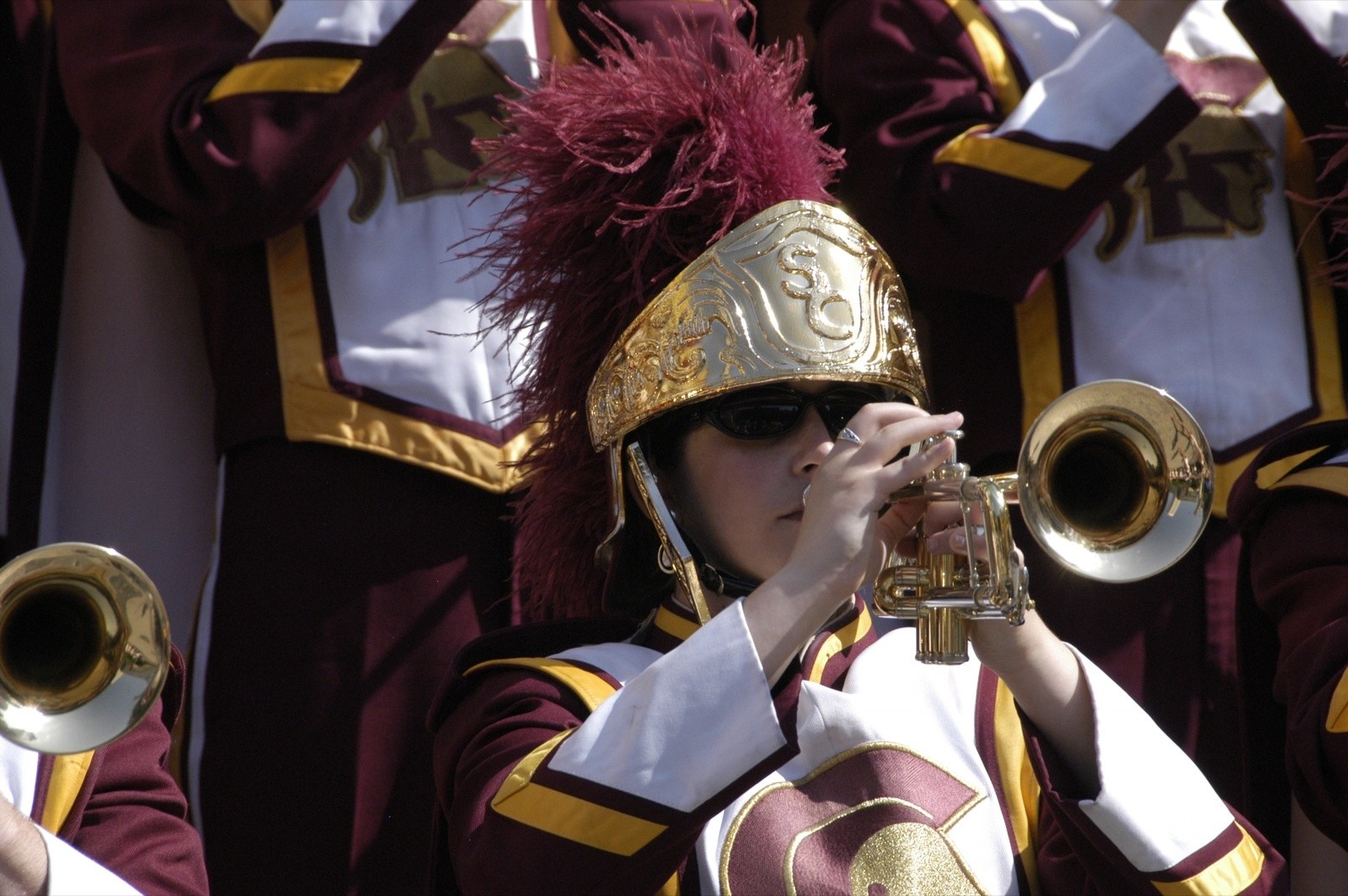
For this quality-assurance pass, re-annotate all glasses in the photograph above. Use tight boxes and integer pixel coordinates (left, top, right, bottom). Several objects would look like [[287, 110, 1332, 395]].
[[694, 386, 888, 444]]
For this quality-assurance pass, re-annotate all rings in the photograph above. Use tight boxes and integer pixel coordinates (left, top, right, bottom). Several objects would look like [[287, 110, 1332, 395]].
[[837, 427, 862, 445]]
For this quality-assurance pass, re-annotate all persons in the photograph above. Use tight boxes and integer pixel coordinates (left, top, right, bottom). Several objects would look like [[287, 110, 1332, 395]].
[[0, 1, 1347, 896]]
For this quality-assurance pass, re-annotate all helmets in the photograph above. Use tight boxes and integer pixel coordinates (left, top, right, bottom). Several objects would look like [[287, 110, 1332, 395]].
[[461, 15, 938, 632]]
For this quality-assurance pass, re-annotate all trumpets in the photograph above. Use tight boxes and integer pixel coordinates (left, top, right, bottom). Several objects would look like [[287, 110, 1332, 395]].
[[802, 378, 1216, 666], [0, 541, 175, 756]]
